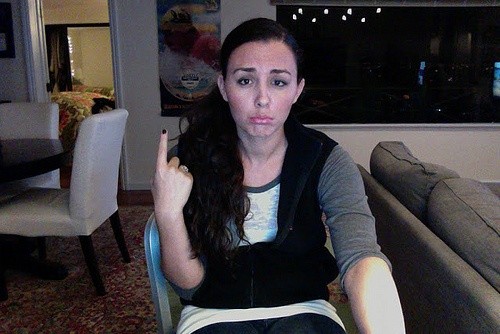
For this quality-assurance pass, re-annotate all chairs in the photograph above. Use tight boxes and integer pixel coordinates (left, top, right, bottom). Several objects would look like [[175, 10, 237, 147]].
[[0, 102, 129, 301]]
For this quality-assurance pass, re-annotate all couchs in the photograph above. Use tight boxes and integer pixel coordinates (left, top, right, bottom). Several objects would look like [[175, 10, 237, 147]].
[[357, 139, 500, 334]]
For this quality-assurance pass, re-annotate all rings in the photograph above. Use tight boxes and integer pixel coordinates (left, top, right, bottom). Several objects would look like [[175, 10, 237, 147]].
[[180, 165, 188, 172]]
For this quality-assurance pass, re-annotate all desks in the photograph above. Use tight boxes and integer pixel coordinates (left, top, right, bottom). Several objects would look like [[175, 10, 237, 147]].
[[0, 136, 68, 183]]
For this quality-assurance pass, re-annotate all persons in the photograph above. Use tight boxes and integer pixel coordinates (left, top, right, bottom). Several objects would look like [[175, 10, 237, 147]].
[[150, 17, 406, 334]]
